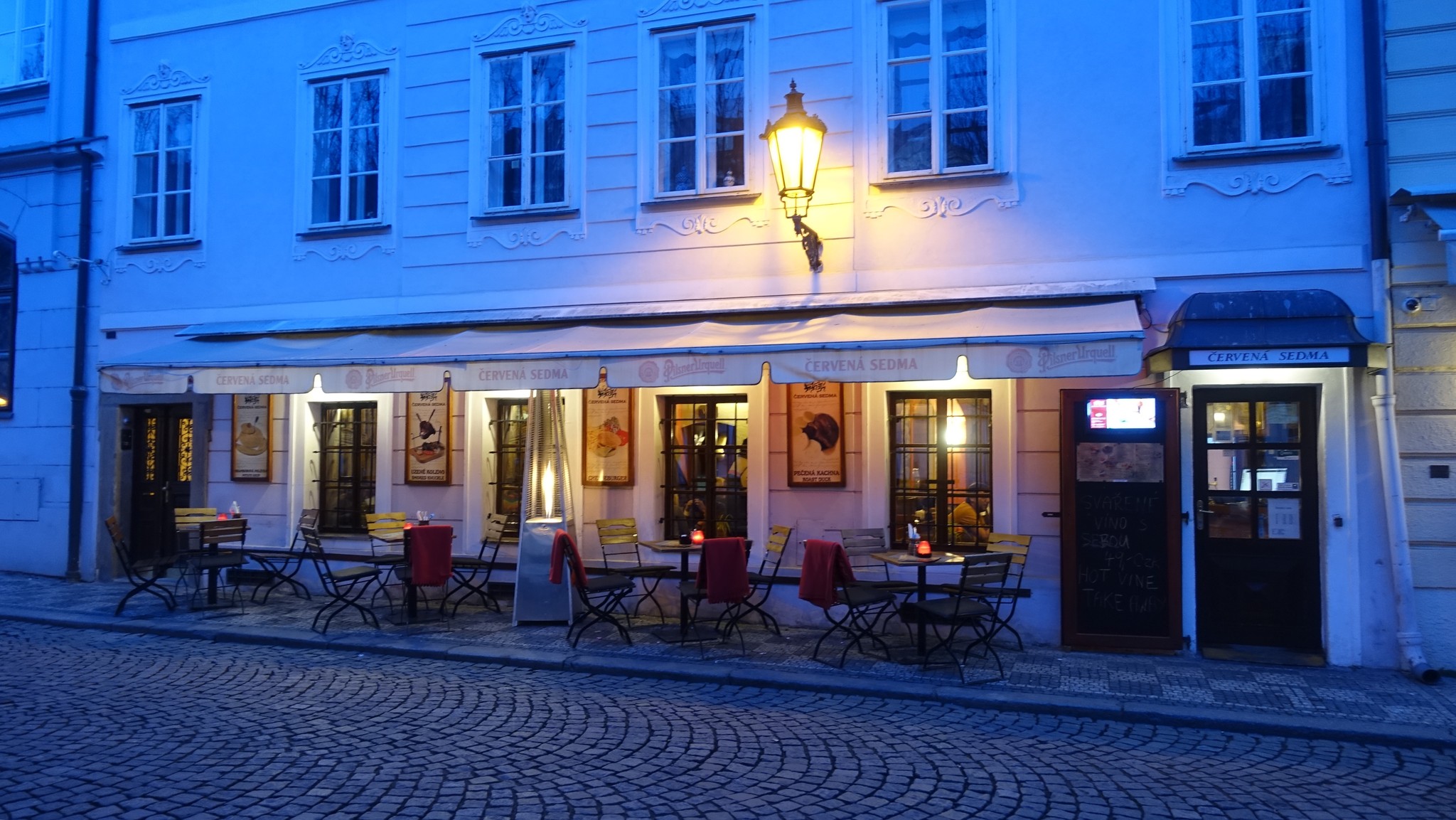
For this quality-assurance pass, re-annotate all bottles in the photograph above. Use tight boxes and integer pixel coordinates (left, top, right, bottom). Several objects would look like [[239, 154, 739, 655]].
[[228, 501, 239, 519]]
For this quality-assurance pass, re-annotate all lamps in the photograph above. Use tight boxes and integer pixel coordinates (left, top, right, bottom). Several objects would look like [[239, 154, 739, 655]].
[[757, 76, 830, 272]]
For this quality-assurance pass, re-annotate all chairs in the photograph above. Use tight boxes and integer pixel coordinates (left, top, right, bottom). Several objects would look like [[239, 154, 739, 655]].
[[837, 526, 919, 650], [298, 523, 384, 635], [103, 515, 181, 614], [715, 523, 792, 643], [437, 511, 510, 618], [948, 532, 1033, 660], [548, 527, 635, 650], [170, 505, 218, 604], [797, 540, 897, 669], [595, 516, 678, 628], [187, 518, 251, 623], [250, 508, 322, 606], [918, 551, 1013, 684], [365, 511, 409, 616], [398, 525, 458, 634], [678, 536, 754, 662]]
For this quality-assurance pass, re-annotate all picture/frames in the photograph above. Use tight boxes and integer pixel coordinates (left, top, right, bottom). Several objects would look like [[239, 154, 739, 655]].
[[581, 372, 636, 488], [229, 393, 274, 485], [403, 376, 453, 486], [784, 377, 846, 489]]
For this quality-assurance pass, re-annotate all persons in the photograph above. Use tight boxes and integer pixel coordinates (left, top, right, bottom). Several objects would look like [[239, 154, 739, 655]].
[[947, 483, 994, 544], [501, 448, 524, 515], [335, 484, 375, 531], [725, 436, 748, 539], [668, 477, 729, 537]]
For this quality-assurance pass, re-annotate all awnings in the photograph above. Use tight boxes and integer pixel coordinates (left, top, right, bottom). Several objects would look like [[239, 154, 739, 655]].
[[97, 294, 1159, 396]]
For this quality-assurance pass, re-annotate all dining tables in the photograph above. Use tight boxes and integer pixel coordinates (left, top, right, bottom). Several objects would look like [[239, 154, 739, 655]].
[[639, 537, 731, 648], [173, 521, 253, 614], [869, 543, 968, 668], [373, 527, 458, 627]]
